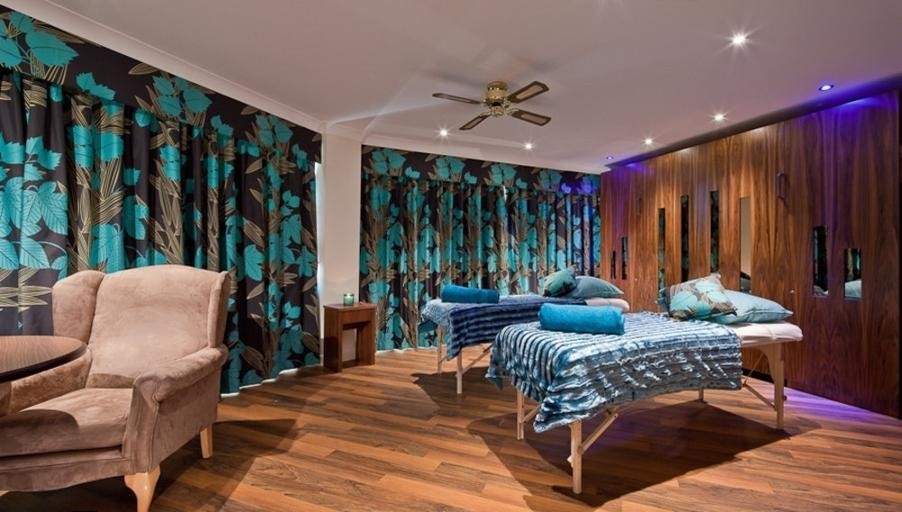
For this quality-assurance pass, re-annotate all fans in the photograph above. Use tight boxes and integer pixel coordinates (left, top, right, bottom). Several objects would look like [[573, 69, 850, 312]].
[[432, 80, 552, 131]]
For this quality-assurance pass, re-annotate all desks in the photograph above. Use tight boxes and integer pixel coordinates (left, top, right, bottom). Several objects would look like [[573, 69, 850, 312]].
[[323, 303, 377, 372], [0, 333, 89, 384]]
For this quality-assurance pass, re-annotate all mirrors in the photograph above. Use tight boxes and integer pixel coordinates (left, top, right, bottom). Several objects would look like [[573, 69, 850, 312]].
[[658, 190, 864, 299], [609, 237, 629, 280]]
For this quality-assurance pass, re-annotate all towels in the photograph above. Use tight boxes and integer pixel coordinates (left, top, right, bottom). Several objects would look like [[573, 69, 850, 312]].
[[441, 285, 501, 304], [536, 301, 626, 334]]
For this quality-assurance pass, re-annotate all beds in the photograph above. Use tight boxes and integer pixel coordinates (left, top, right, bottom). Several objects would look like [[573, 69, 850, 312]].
[[420, 293, 630, 394], [499, 311, 804, 495]]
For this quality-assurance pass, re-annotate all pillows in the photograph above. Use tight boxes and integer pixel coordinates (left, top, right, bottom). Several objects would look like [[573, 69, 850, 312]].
[[653, 271, 794, 324], [537, 263, 625, 300]]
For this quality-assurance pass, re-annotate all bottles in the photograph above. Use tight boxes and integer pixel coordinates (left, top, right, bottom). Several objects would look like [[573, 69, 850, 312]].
[[343, 292, 355, 306]]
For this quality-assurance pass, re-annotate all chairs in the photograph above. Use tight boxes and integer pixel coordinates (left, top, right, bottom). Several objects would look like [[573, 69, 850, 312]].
[[0, 263, 231, 512]]
